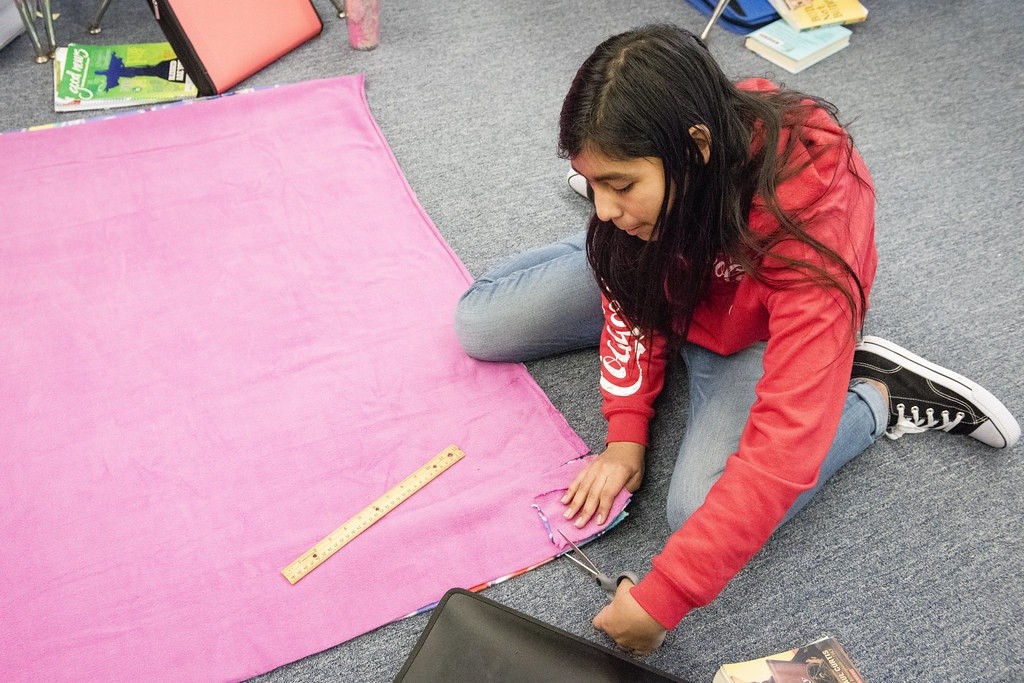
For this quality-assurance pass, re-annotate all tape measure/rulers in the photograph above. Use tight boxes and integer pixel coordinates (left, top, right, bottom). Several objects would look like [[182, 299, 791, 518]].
[[280, 443, 466, 585]]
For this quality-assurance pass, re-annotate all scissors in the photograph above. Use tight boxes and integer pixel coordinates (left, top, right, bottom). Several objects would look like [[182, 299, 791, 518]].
[[556, 528, 639, 603]]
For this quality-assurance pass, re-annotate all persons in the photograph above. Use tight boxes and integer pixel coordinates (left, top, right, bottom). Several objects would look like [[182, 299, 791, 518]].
[[454, 22, 1020, 658]]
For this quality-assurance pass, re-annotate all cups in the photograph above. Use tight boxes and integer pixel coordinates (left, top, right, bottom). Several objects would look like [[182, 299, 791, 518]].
[[344, 0, 381, 50]]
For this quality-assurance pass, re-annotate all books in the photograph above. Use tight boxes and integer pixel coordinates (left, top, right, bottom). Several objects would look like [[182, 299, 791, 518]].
[[712, 636, 866, 683], [762, 0, 871, 32], [743, 17, 855, 74], [50, 39, 199, 113]]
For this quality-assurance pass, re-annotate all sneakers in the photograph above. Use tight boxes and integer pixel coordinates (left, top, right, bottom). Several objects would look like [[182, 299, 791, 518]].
[[568, 169, 594, 206], [851, 336, 1021, 451]]
